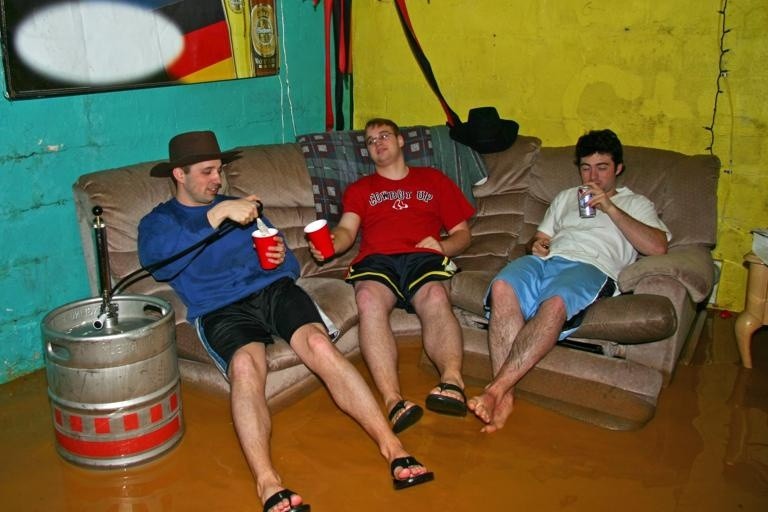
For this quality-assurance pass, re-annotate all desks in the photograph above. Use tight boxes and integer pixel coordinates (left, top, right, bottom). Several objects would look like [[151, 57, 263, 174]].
[[734, 252, 767, 371]]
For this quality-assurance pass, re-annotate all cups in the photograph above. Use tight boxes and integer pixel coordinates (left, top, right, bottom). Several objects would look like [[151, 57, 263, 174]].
[[250, 227, 282, 270], [303, 219, 336, 261]]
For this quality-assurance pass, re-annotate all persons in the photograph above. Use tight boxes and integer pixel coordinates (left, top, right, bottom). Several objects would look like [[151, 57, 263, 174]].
[[466, 128, 672, 434], [307, 119, 478, 435], [137, 129, 435, 510]]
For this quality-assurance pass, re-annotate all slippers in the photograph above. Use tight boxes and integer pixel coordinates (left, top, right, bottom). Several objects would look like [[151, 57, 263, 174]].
[[390, 455, 434, 490], [426, 383, 468, 417], [389, 399, 424, 432], [262, 489, 311, 511]]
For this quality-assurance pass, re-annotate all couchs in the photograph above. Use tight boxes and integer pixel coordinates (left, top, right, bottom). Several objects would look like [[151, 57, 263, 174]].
[[417, 142, 720, 431], [72, 161, 342, 416], [232, 120, 540, 354]]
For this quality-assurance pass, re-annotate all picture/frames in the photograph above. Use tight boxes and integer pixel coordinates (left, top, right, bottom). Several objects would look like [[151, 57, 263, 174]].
[[1, 0, 280, 100]]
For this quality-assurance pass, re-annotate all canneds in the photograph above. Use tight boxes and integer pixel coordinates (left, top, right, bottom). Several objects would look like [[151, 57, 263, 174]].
[[578, 183, 597, 219]]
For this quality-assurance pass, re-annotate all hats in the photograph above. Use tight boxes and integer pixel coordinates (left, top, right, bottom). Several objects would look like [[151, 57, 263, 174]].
[[449, 106, 519, 152], [149, 130, 243, 178]]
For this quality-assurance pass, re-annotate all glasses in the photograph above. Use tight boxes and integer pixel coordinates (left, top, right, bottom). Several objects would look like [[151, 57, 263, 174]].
[[367, 133, 395, 145]]
